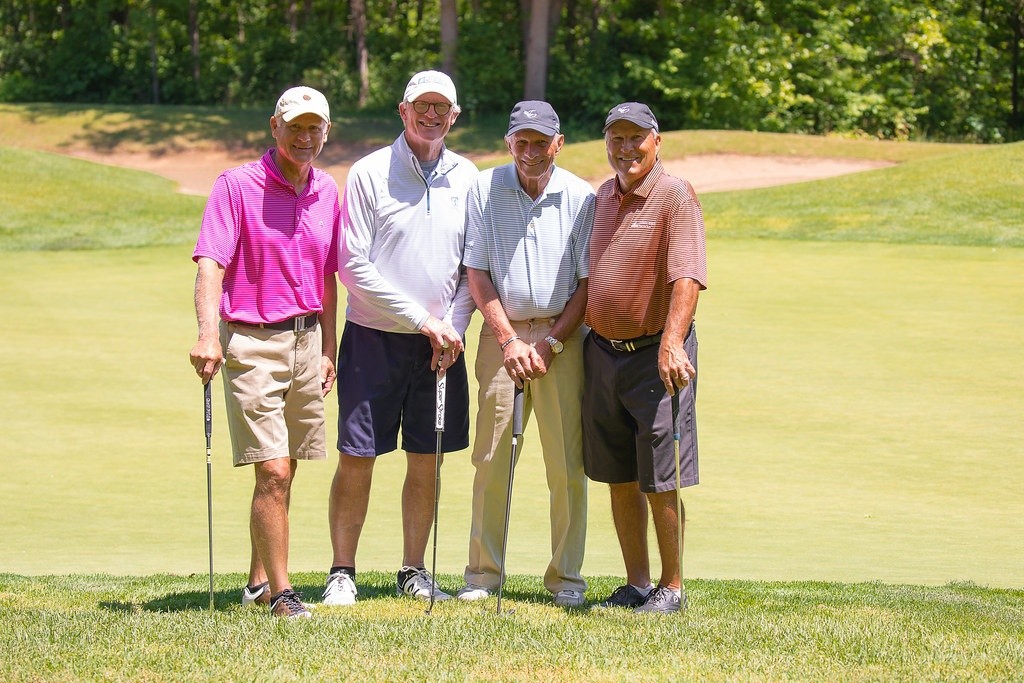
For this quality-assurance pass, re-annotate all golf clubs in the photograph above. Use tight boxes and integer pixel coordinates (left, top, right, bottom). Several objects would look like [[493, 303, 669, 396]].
[[204, 379, 216, 611], [424, 351, 446, 615], [497, 377, 524, 616], [671, 378, 684, 615]]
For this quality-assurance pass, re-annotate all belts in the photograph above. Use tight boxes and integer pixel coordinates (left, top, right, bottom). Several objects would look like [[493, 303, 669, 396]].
[[232, 314, 318, 332], [591, 329, 662, 352]]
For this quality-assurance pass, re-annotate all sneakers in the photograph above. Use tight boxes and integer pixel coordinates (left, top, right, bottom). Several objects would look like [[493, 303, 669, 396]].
[[322, 566, 358, 605], [242, 581, 270, 607], [598, 584, 647, 609], [396, 566, 452, 602], [632, 585, 688, 613], [269, 587, 311, 620]]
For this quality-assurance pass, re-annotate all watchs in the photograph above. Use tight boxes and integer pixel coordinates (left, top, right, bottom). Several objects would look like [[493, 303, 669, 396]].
[[544, 335, 565, 355]]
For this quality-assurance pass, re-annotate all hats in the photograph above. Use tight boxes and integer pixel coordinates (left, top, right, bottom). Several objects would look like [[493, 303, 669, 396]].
[[507, 101, 560, 136], [404, 70, 461, 114], [275, 86, 330, 127], [601, 102, 659, 133]]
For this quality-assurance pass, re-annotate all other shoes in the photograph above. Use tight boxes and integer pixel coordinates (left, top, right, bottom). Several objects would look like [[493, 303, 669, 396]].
[[457, 583, 492, 601], [554, 590, 585, 607]]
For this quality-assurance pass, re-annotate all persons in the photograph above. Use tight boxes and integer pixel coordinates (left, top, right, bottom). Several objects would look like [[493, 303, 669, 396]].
[[189, 86, 340, 619], [321, 68, 481, 609], [455, 100, 597, 607], [581, 102, 708, 615]]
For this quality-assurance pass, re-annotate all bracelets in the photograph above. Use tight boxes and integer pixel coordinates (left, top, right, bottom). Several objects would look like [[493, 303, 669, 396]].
[[498, 336, 520, 352]]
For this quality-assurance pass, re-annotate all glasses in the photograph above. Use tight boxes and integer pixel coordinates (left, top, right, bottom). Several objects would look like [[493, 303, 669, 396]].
[[411, 100, 452, 116]]
